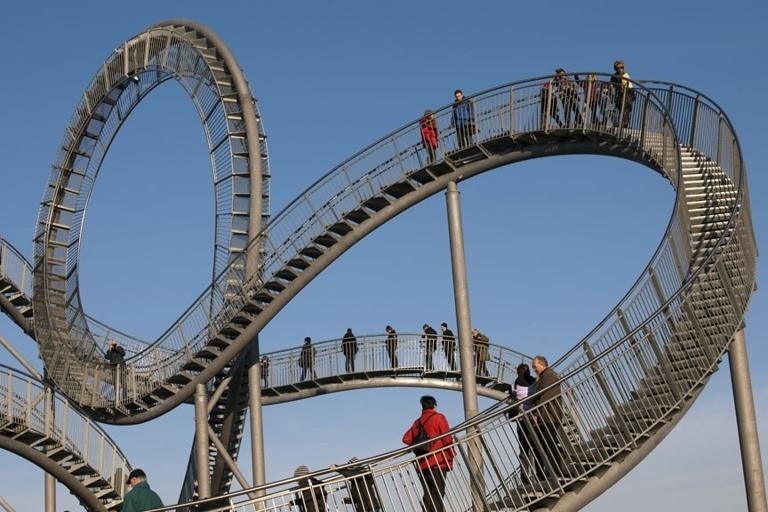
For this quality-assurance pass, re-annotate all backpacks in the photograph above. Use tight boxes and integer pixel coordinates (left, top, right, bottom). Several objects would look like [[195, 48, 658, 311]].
[[413, 414, 436, 454]]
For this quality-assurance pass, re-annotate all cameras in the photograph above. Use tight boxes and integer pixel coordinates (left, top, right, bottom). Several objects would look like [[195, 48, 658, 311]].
[[112, 343, 117, 348]]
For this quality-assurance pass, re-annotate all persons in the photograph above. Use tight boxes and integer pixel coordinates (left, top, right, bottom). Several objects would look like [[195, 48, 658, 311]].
[[341, 328, 360, 372], [450, 90, 476, 149], [328, 455, 380, 512], [122, 468, 167, 511], [440, 322, 457, 370], [287, 464, 328, 512], [102, 338, 129, 402], [472, 326, 491, 377], [418, 323, 438, 371], [537, 61, 637, 129], [299, 336, 318, 382], [533, 354, 563, 478], [385, 325, 399, 368], [420, 109, 440, 165], [402, 396, 458, 511], [259, 354, 270, 388], [509, 363, 546, 484]]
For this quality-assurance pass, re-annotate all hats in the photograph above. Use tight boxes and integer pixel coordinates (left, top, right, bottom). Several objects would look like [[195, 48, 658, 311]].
[[347, 456, 362, 469], [125, 469, 146, 484], [293, 465, 309, 478]]
